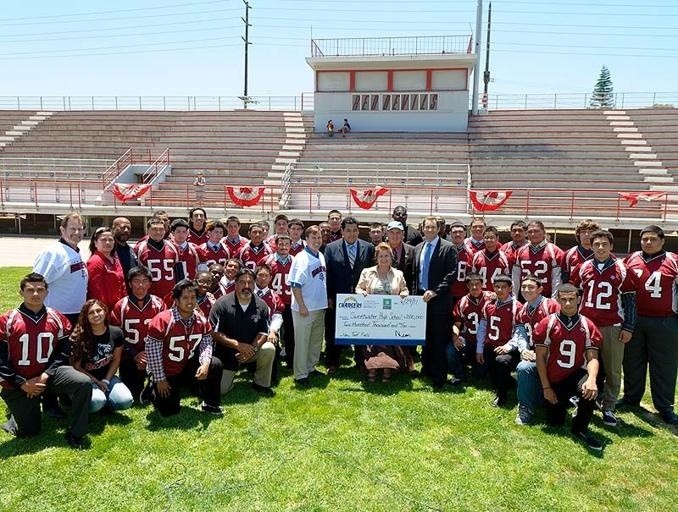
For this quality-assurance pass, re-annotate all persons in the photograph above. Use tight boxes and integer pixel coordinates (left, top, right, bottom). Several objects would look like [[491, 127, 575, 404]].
[[193, 172, 206, 206], [0, 208, 678, 450], [326, 120, 335, 137], [338, 119, 351, 137]]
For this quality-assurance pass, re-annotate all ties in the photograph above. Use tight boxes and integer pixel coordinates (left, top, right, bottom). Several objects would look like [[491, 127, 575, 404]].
[[348, 245, 356, 269], [422, 243, 432, 289]]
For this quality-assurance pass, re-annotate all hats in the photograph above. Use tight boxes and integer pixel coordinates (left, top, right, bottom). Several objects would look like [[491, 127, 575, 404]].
[[387, 221, 404, 230]]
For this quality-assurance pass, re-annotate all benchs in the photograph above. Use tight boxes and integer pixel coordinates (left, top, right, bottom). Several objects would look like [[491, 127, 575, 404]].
[[1, 109, 677, 222]]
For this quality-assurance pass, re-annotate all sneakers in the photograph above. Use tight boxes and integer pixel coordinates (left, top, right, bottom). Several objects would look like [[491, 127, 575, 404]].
[[368, 373, 376, 382], [140, 383, 150, 404], [602, 407, 617, 426], [660, 411, 678, 424], [615, 399, 639, 410], [251, 381, 273, 397], [382, 374, 391, 382], [300, 377, 309, 386], [494, 391, 506, 405], [65, 430, 92, 447], [449, 377, 467, 384], [202, 401, 224, 414], [519, 404, 535, 422], [571, 426, 602, 451]]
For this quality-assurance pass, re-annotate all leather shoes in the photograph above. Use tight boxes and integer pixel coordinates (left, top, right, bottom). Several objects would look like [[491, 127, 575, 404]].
[[60, 395, 72, 406], [47, 404, 67, 418]]
